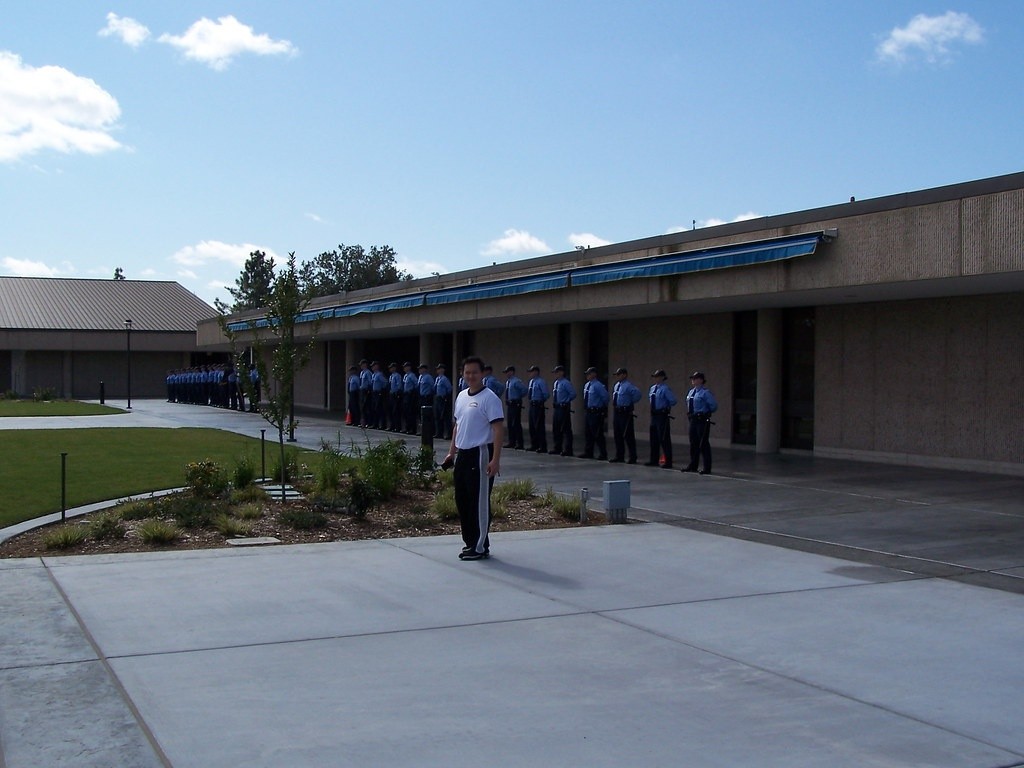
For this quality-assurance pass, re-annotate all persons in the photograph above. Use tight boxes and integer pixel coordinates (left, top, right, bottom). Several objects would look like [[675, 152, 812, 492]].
[[443, 357, 504, 561], [166, 363, 261, 413], [459, 362, 718, 474], [348, 359, 452, 439]]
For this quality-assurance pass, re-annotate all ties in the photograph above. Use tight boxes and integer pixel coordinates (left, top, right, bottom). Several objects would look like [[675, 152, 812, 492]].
[[347, 371, 443, 394], [459, 378, 463, 390], [483, 379, 696, 415]]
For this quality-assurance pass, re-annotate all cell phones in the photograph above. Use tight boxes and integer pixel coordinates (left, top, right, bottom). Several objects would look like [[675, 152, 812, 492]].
[[440, 458, 454, 473]]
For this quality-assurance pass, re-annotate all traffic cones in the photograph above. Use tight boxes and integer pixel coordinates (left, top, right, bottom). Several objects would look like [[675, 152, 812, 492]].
[[344, 408, 353, 426]]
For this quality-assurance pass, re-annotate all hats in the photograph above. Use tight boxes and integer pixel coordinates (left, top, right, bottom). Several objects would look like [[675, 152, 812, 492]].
[[651, 369, 665, 377], [551, 365, 565, 373], [168, 364, 224, 374], [527, 365, 537, 372], [502, 366, 515, 374], [584, 367, 596, 374], [483, 366, 492, 370], [248, 364, 255, 369], [689, 372, 704, 379], [613, 368, 627, 376], [347, 360, 447, 372]]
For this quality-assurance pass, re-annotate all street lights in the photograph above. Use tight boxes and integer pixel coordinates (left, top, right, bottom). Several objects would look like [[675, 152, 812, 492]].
[[123, 317, 136, 409]]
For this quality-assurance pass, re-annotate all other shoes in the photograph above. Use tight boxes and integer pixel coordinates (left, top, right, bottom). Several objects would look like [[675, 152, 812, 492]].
[[503, 443, 710, 475], [462, 547, 471, 552], [167, 399, 257, 413], [459, 552, 489, 560], [346, 423, 452, 442]]
